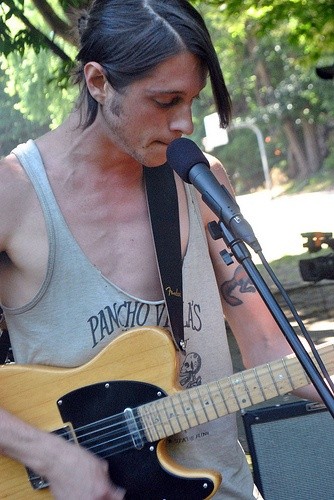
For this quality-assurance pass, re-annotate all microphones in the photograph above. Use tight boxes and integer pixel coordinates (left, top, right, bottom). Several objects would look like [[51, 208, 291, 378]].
[[166, 137, 260, 251]]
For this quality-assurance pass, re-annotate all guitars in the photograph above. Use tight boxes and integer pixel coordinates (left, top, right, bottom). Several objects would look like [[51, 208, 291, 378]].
[[0, 323, 334, 499]]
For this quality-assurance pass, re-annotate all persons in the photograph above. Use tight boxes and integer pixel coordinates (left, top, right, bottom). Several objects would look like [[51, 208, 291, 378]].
[[0, 1, 334, 500]]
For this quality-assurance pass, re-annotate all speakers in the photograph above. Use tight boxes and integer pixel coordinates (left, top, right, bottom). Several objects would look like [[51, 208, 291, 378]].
[[242, 393, 334, 500]]
